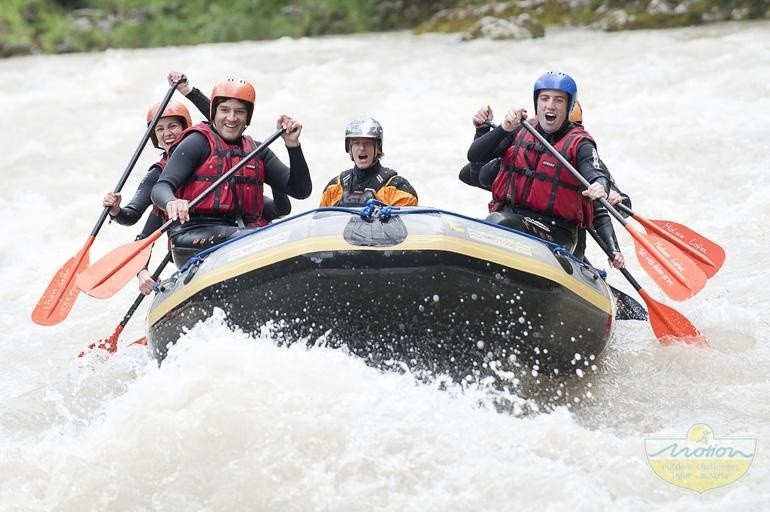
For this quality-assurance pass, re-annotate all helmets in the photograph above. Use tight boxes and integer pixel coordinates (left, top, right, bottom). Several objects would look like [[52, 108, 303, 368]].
[[345, 116, 383, 158], [534, 72, 582, 123], [147, 99, 192, 149], [210, 77, 255, 125]]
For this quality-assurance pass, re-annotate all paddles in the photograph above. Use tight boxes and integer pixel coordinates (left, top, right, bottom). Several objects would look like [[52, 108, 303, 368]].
[[75, 128, 299, 298], [69, 253, 174, 362], [586, 225, 710, 347], [520, 116, 707, 302], [32, 74, 187, 327], [481, 115, 725, 278]]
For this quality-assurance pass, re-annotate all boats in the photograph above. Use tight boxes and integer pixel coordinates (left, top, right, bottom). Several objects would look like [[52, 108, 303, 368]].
[[145, 198, 615, 377]]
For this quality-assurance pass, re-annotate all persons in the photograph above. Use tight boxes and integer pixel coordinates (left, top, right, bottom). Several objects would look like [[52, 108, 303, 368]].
[[151, 78, 313, 270], [466, 70, 610, 256], [134, 186, 297, 297], [458, 160, 626, 273], [471, 99, 633, 220], [319, 115, 419, 211], [100, 70, 215, 227]]
